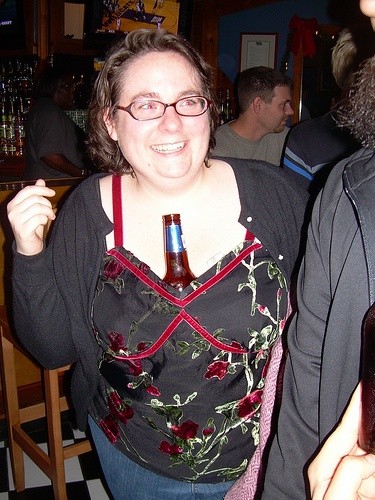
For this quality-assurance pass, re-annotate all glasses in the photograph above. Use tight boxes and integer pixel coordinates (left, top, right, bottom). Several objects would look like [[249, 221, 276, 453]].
[[112, 96, 211, 122]]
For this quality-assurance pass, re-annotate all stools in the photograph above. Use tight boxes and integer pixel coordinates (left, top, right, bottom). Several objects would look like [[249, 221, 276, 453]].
[[0, 305, 92, 500]]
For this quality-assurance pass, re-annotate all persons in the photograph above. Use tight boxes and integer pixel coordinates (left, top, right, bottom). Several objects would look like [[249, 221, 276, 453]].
[[20, 67, 93, 180], [6, 28, 320, 500], [210, 0, 375, 500]]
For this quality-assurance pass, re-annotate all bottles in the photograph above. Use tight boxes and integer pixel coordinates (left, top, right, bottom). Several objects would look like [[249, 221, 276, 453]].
[[0, 56, 36, 163], [357, 301, 375, 454], [162, 213, 196, 292], [218, 88, 234, 125]]
